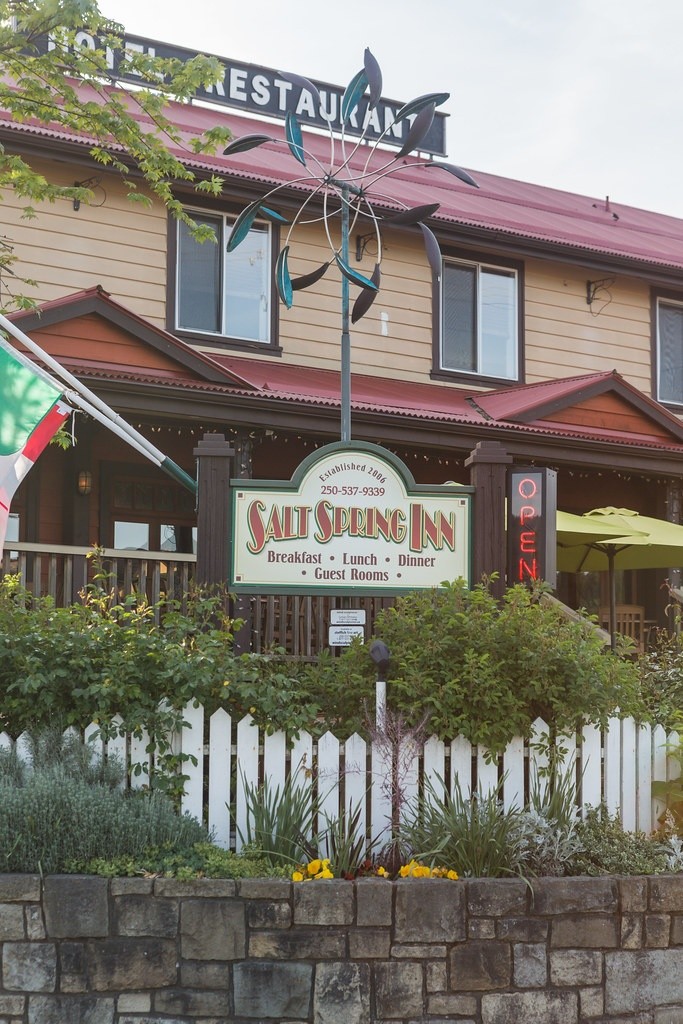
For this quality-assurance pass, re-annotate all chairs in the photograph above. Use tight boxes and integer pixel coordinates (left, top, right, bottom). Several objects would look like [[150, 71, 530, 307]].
[[598, 605, 645, 651], [260, 595, 307, 655]]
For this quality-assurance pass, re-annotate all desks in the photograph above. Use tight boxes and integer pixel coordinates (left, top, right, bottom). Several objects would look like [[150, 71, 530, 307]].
[[593, 619, 658, 652]]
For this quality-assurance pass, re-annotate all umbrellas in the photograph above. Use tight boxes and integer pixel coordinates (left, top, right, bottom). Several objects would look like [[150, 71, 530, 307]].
[[444, 480, 650, 549], [555, 507, 683, 655]]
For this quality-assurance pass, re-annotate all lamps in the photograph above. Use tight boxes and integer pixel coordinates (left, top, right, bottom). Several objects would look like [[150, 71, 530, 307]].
[[76, 469, 93, 496]]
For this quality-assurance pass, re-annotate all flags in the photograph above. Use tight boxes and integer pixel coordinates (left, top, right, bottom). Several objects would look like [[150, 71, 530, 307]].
[[0, 337, 73, 561]]
